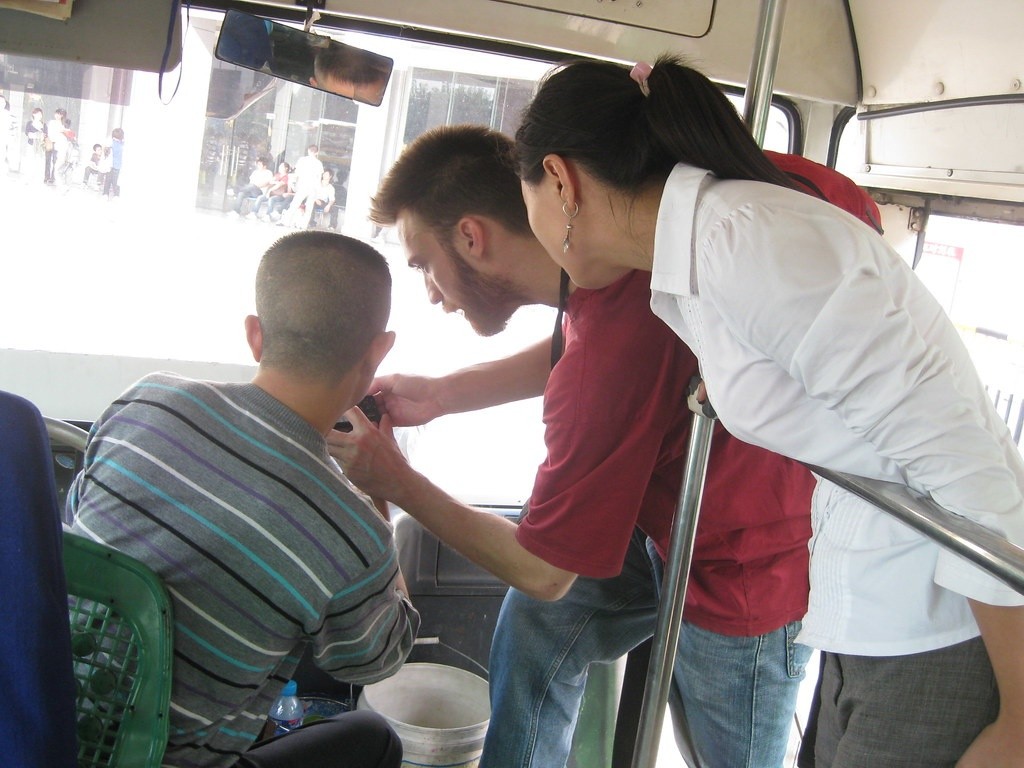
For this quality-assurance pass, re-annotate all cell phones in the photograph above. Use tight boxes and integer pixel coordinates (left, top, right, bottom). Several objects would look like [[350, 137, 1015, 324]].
[[333, 394, 380, 428]]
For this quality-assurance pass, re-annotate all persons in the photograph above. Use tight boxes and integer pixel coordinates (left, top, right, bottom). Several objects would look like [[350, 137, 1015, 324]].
[[510, 55, 1024, 768], [0, 97, 340, 237], [328, 120, 819, 768], [62, 232, 421, 768], [304, 46, 394, 106]]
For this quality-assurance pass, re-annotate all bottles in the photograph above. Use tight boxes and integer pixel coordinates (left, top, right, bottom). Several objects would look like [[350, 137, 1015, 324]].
[[262, 680, 304, 742]]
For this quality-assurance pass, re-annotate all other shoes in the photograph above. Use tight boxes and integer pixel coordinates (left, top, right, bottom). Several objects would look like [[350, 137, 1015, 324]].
[[246, 212, 257, 219], [261, 215, 271, 221], [227, 210, 239, 219]]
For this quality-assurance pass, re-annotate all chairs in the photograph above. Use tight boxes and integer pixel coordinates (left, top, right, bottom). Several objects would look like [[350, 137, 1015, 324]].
[[0, 389, 170, 768]]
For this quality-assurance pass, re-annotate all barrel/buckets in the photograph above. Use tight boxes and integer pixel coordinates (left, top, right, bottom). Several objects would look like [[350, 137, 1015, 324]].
[[351, 636, 492, 768]]
